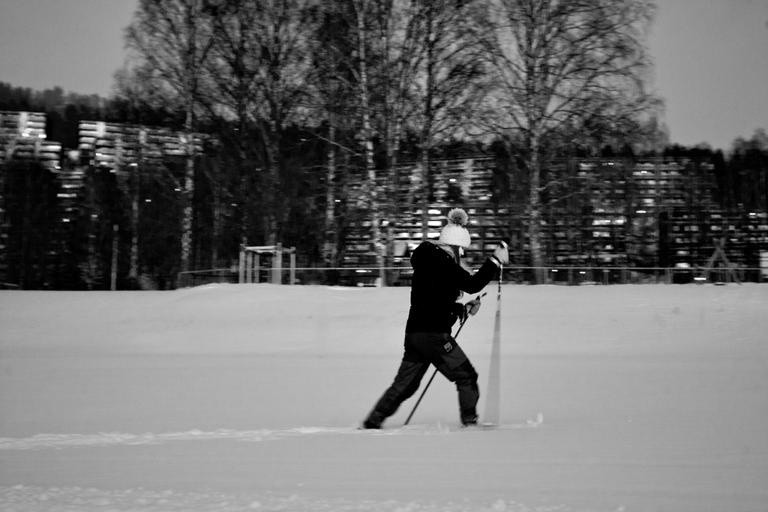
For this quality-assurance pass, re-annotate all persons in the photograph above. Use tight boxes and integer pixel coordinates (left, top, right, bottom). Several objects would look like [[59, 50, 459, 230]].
[[362, 208, 509, 429]]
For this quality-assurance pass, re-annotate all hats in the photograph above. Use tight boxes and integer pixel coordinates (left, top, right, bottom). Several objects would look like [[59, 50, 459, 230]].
[[439, 206, 471, 249]]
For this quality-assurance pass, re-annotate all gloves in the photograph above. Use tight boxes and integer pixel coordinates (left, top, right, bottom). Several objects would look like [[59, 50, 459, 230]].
[[490, 240, 510, 269], [466, 299, 481, 315]]
